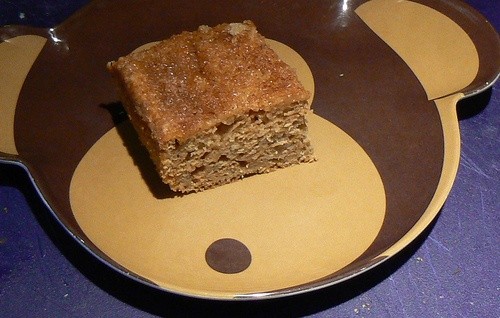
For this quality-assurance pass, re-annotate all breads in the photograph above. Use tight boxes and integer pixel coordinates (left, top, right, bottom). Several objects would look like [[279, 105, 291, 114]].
[[105, 20, 317, 194]]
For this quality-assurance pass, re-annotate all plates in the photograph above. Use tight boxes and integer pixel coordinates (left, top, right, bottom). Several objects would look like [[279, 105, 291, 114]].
[[0, 0, 500, 301]]
